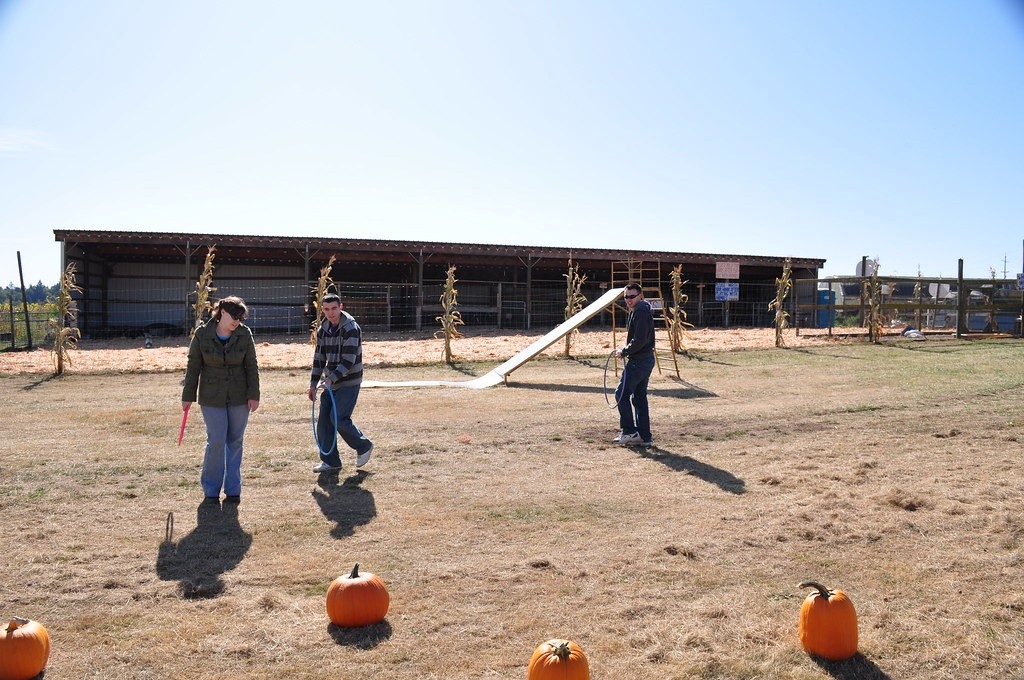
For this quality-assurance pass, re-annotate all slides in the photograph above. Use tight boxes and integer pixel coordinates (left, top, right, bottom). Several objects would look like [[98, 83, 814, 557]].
[[490, 287, 624, 375]]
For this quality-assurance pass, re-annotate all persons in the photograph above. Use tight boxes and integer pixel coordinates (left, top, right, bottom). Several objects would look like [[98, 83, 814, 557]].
[[181, 296, 260, 503], [614, 284, 656, 442], [307, 291, 373, 472]]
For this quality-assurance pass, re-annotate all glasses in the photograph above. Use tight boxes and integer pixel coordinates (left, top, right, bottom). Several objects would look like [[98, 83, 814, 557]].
[[624, 293, 639, 299], [223, 308, 244, 321]]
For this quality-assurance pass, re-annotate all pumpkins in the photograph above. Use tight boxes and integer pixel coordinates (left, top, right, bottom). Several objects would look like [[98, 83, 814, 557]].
[[798, 580, 858, 661], [326, 562, 389, 626], [0, 620, 49, 680], [528, 638, 590, 680]]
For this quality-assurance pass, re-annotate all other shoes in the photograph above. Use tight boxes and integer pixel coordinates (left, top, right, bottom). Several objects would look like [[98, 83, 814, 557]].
[[206, 497, 219, 504], [226, 495, 240, 503]]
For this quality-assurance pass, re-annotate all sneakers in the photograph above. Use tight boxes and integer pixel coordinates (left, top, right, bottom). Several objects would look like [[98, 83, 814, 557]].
[[313, 462, 342, 472], [620, 433, 653, 446], [613, 431, 640, 443], [356, 445, 373, 466]]
